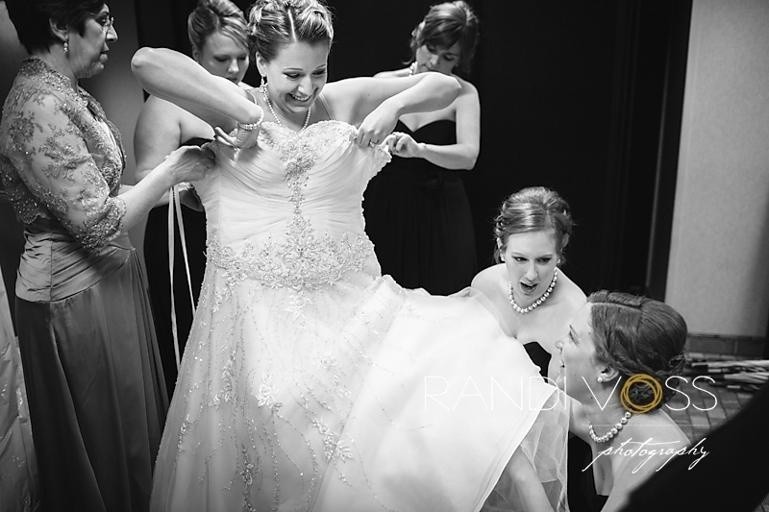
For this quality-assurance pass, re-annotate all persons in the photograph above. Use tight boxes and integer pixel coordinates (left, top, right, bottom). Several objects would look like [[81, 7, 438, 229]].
[[0, 0, 692, 512]]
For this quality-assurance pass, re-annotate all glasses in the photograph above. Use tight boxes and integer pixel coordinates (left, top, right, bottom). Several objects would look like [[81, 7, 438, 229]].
[[86, 11, 114, 29]]
